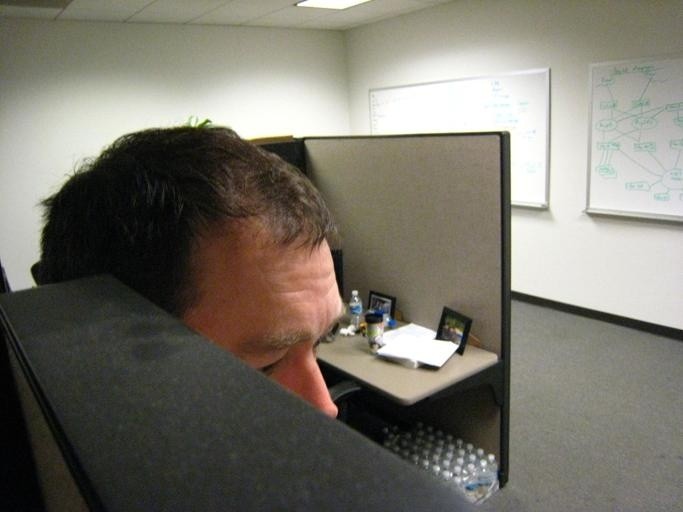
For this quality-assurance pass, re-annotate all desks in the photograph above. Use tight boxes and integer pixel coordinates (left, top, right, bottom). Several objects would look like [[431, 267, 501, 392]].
[[316, 303, 505, 490]]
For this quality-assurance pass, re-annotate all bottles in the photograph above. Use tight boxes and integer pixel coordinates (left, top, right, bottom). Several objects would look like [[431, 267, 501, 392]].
[[369, 420, 502, 502], [348, 290, 363, 331]]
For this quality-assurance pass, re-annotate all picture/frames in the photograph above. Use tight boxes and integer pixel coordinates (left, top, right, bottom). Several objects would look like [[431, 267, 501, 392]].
[[367, 290, 395, 322], [435, 306, 472, 355]]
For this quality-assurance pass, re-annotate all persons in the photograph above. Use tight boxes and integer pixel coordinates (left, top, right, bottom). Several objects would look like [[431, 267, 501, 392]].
[[34, 116, 349, 422]]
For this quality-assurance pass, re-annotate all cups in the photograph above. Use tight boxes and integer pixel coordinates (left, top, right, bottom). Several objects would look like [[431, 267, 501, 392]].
[[364, 312, 385, 354]]
